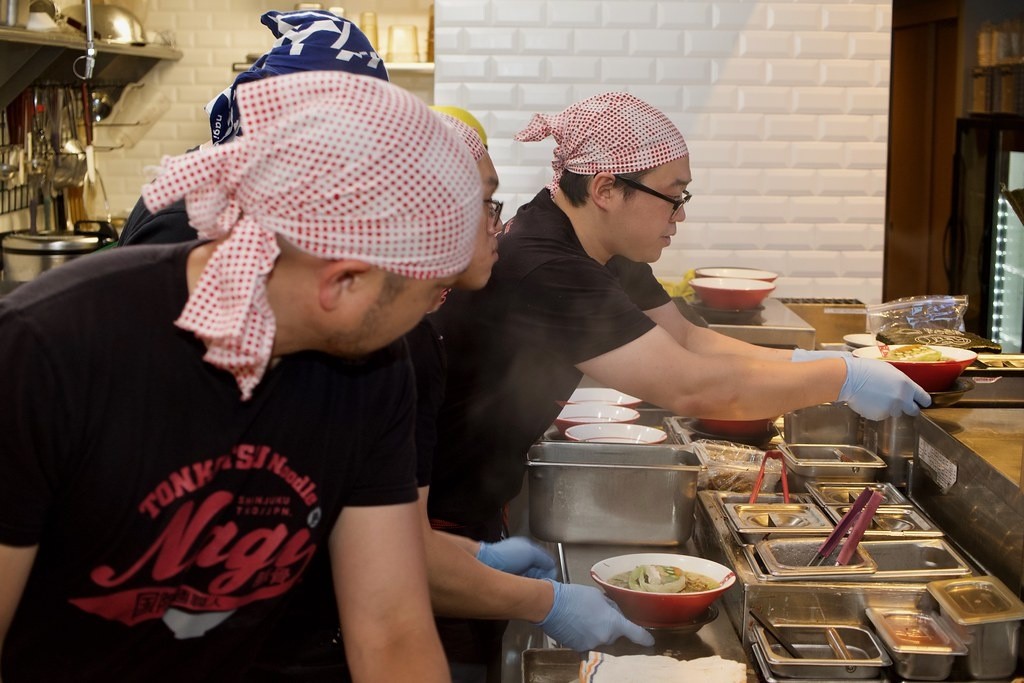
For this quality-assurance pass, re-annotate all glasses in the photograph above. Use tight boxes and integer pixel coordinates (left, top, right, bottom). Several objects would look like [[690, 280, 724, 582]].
[[483, 199, 503, 228], [593, 171, 692, 220]]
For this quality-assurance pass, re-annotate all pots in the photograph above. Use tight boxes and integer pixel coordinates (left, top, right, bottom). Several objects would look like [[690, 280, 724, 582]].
[[4, 221, 118, 282]]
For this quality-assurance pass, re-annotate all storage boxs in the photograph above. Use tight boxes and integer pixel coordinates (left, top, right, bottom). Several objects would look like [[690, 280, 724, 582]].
[[864, 607, 968, 681], [741, 538, 972, 583], [805, 480, 912, 510], [755, 538, 877, 577], [723, 501, 836, 539], [751, 623, 892, 679], [776, 442, 888, 480], [824, 505, 948, 540], [926, 575, 1024, 681], [521, 440, 709, 550]]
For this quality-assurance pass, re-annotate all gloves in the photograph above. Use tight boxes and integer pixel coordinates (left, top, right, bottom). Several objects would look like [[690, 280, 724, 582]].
[[474, 539, 557, 583], [837, 355, 932, 420], [792, 349, 854, 364], [533, 578, 656, 653]]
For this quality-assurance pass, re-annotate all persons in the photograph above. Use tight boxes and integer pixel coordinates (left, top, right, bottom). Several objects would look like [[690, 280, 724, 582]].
[[427, 92, 931, 682], [0, 10, 653, 682]]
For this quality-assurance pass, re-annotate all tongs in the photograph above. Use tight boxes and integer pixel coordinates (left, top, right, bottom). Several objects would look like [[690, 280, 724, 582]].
[[748, 451, 790, 504], [807, 487, 882, 567]]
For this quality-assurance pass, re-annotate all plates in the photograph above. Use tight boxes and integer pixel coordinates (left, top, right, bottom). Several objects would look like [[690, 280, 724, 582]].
[[843, 334, 885, 348]]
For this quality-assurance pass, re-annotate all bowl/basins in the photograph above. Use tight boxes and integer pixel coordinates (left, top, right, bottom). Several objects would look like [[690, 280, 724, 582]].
[[554, 405, 640, 436], [566, 422, 667, 444], [567, 388, 643, 407], [58, 4, 147, 44], [695, 267, 778, 283], [688, 278, 776, 309], [852, 345, 978, 391], [591, 553, 736, 622]]
